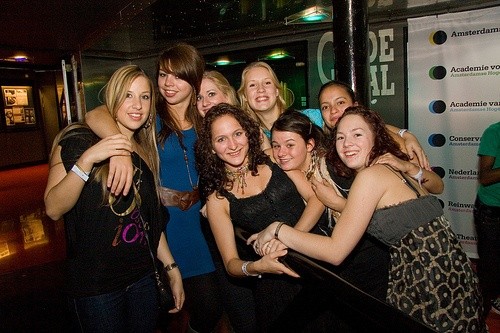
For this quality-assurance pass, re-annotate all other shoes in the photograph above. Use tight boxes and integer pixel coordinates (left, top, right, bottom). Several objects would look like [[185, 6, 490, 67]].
[[490, 299, 500, 316]]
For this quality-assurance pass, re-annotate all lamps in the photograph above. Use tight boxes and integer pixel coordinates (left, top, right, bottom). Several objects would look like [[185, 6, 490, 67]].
[[206, 54, 248, 67], [284, 1, 333, 26], [259, 45, 299, 61]]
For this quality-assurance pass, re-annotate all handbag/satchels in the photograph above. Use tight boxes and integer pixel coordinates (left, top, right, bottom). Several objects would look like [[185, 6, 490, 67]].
[[157, 269, 187, 328]]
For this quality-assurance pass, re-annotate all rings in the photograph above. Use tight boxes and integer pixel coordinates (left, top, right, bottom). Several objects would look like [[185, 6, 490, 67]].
[[267, 245, 271, 248], [256, 244, 260, 248]]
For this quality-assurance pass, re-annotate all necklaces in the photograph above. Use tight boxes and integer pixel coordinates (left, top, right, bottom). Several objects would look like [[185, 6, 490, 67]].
[[177, 126, 201, 190], [224, 163, 252, 196]]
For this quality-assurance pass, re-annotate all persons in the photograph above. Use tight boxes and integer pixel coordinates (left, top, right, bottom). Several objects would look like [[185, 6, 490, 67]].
[[474, 118, 500, 333], [43, 44, 485, 333]]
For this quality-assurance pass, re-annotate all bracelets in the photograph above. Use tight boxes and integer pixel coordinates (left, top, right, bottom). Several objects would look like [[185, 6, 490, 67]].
[[274, 221, 285, 241], [71, 165, 90, 183], [241, 260, 262, 280], [164, 262, 178, 273], [398, 128, 407, 137], [411, 167, 423, 181]]
